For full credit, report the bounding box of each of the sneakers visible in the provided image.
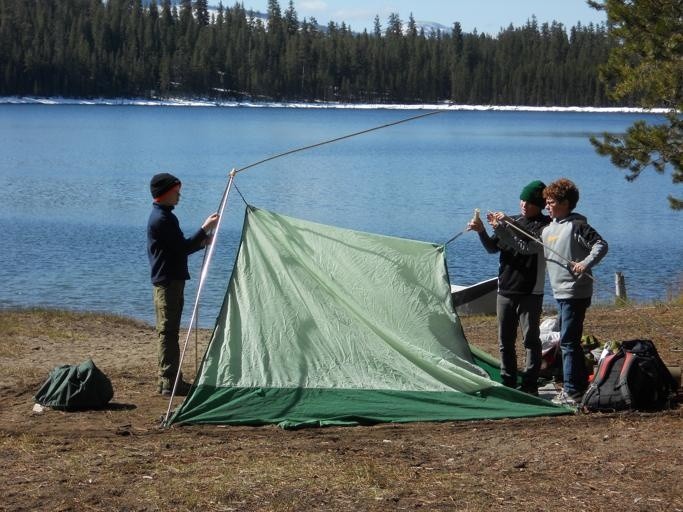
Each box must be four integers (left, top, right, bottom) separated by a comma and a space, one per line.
517, 385, 539, 396
551, 390, 581, 405
157, 380, 193, 396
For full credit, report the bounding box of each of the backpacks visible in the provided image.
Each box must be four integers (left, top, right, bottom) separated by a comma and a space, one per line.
582, 348, 661, 411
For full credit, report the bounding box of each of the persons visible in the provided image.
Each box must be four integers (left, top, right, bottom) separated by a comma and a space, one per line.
485, 178, 609, 405
464, 180, 546, 397
147, 172, 218, 396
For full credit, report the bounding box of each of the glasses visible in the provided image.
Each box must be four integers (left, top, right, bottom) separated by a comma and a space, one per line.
544, 201, 555, 207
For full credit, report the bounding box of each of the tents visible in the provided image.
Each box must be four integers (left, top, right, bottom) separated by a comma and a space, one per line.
167, 203, 575, 424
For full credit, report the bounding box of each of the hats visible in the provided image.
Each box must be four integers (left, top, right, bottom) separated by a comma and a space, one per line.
150, 172, 181, 203
520, 179, 547, 209
